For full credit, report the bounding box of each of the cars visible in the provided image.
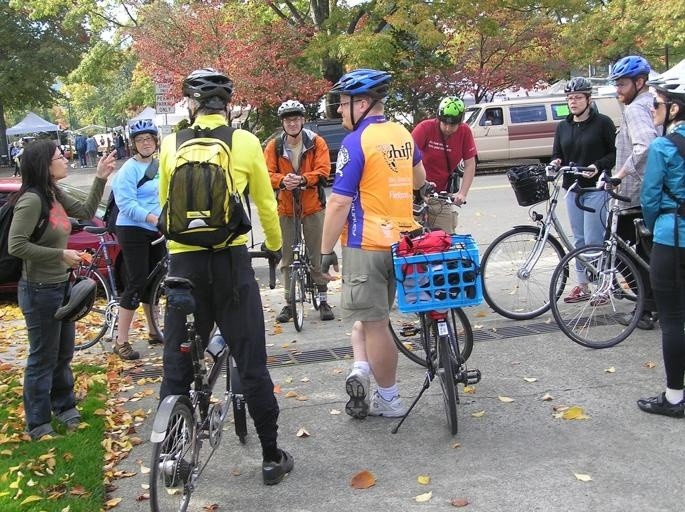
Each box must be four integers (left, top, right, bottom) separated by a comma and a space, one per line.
17, 136, 38, 147
61, 143, 78, 159
0, 179, 129, 302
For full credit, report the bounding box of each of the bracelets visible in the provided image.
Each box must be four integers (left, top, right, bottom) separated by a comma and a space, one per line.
302, 176, 305, 189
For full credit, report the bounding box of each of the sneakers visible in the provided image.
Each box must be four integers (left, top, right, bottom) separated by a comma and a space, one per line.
66, 417, 81, 433
369, 390, 407, 418
276, 305, 293, 323
616, 309, 654, 330
589, 288, 610, 306
32, 431, 55, 440
345, 371, 370, 419
564, 285, 591, 303
320, 303, 334, 321
637, 392, 684, 418
262, 448, 294, 485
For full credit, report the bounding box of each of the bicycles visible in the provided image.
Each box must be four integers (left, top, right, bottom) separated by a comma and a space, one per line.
67, 224, 219, 353
377, 188, 484, 436
274, 180, 321, 331
549, 167, 650, 349
481, 161, 639, 323
413, 190, 467, 230
148, 313, 247, 512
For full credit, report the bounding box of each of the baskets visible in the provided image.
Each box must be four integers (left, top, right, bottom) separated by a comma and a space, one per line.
390, 233, 484, 314
506, 163, 550, 206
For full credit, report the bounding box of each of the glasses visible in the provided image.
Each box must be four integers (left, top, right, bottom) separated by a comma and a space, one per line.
134, 136, 153, 143
565, 96, 586, 101
653, 96, 673, 109
613, 82, 624, 89
283, 116, 301, 124
52, 154, 64, 162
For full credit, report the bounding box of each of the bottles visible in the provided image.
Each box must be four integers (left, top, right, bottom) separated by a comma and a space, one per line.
203, 327, 227, 360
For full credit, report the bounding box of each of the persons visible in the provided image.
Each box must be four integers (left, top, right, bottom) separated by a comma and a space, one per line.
7, 140, 118, 440
603, 56, 661, 328
262, 100, 335, 323
549, 77, 616, 307
157, 67, 293, 486
412, 96, 477, 234
110, 118, 167, 359
635, 73, 685, 420
319, 69, 426, 418
9, 125, 125, 176
487, 111, 502, 125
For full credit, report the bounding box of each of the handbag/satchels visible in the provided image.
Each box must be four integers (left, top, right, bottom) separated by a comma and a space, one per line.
447, 172, 460, 193
103, 190, 119, 234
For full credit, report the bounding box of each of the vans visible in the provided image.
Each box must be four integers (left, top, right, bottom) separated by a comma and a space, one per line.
456, 96, 625, 174
260, 117, 346, 173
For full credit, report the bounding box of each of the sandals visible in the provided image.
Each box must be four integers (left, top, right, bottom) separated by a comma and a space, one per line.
148, 333, 162, 344
114, 342, 138, 360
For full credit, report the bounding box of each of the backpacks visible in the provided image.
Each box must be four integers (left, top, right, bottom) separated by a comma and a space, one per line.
0, 187, 50, 284
156, 124, 253, 310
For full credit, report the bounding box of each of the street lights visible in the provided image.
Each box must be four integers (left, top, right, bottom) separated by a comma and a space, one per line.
103, 113, 109, 135
65, 91, 72, 131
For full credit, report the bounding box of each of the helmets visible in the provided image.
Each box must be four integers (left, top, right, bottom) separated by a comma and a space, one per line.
564, 76, 592, 93
129, 119, 158, 138
53, 275, 97, 323
645, 74, 685, 95
436, 95, 465, 124
183, 68, 234, 101
278, 99, 306, 116
328, 68, 397, 98
606, 55, 650, 81
